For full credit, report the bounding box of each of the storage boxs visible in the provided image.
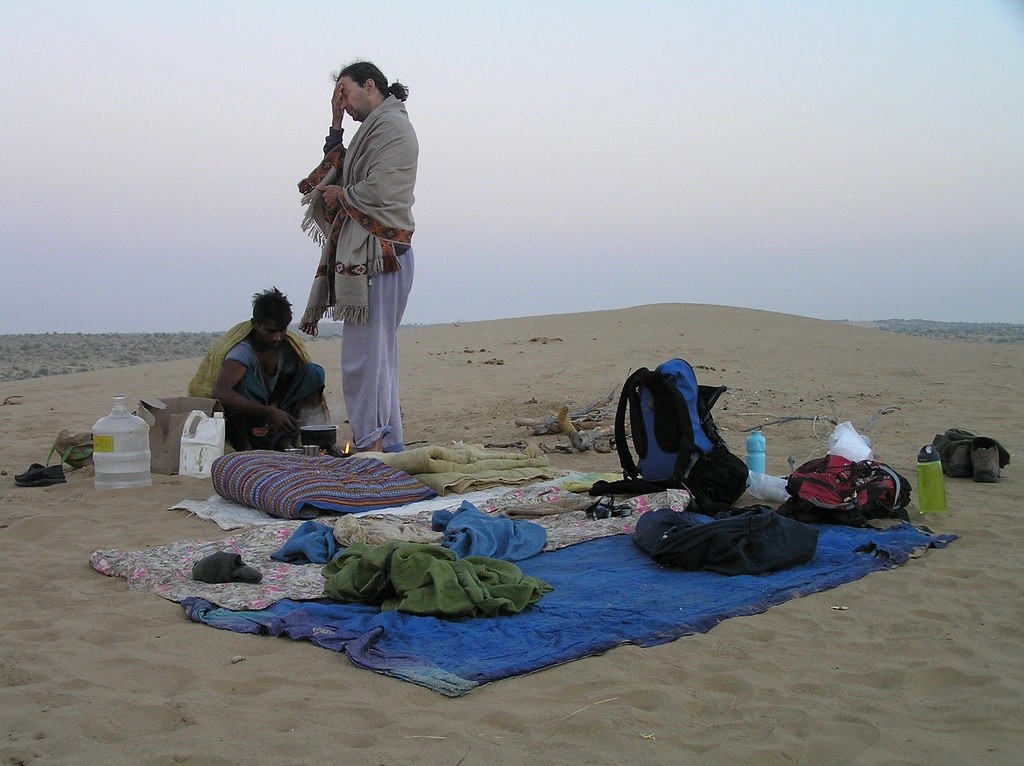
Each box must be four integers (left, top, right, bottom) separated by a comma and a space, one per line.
137, 393, 227, 476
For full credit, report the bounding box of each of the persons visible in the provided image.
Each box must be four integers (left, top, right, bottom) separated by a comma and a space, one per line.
186, 286, 325, 452
315, 60, 419, 457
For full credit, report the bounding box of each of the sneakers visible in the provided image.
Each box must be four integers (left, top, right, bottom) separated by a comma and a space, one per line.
940, 437, 999, 483
14, 463, 66, 487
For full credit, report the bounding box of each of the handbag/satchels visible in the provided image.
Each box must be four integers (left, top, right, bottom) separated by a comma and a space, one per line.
46, 429, 94, 470
673, 444, 749, 517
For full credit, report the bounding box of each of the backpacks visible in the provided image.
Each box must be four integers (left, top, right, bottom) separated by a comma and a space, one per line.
775, 496, 910, 531
786, 455, 912, 511
615, 358, 730, 481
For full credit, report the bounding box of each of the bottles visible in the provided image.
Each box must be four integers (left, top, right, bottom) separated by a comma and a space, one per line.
916, 445, 947, 514
744, 430, 766, 486
178, 410, 227, 481
90, 395, 152, 492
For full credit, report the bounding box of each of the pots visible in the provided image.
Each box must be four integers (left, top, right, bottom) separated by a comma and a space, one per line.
284, 445, 320, 457
299, 424, 338, 448
